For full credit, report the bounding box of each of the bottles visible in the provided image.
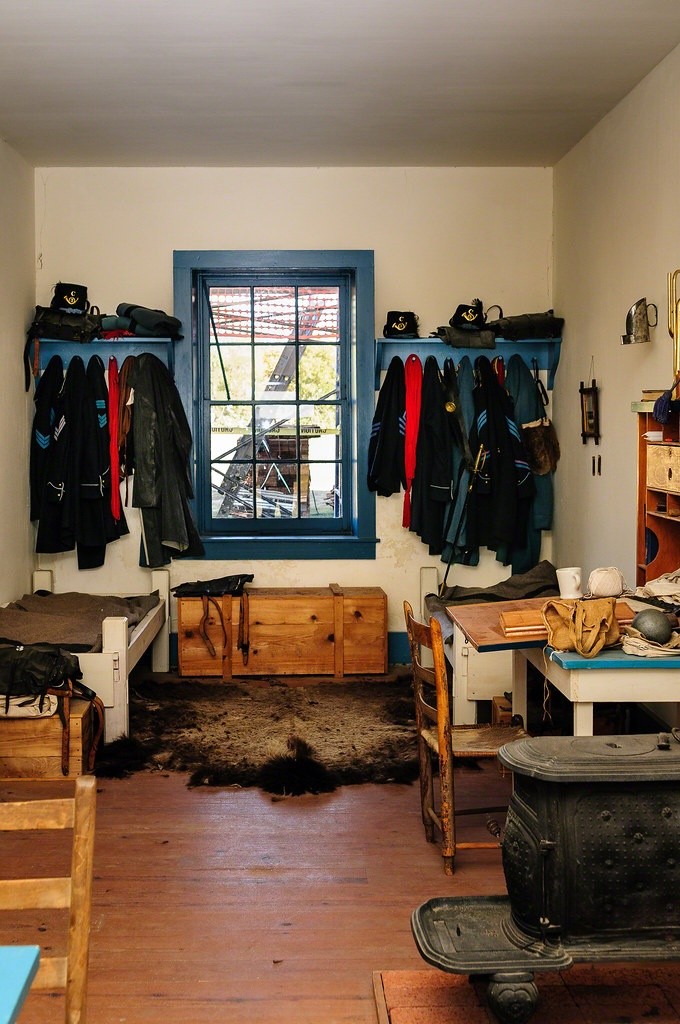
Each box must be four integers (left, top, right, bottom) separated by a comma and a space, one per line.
589, 568, 623, 597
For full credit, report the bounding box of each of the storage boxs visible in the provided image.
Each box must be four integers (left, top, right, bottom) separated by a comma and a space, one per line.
174, 584, 388, 677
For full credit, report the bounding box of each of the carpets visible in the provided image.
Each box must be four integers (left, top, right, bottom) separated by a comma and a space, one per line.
92, 676, 483, 797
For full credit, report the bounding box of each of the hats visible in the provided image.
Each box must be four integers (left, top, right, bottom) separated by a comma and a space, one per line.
383, 310, 420, 338
50, 282, 88, 313
449, 303, 484, 331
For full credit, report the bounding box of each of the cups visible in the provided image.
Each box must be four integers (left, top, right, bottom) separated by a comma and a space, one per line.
556, 567, 583, 600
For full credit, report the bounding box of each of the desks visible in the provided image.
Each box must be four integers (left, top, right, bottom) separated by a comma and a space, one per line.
510, 645, 680, 737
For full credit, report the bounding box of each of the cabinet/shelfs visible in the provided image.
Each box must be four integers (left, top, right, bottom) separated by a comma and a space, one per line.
636, 411, 680, 586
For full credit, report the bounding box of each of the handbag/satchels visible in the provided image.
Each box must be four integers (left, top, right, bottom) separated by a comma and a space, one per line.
27, 303, 106, 342
0, 645, 97, 730
541, 597, 622, 658
483, 305, 564, 340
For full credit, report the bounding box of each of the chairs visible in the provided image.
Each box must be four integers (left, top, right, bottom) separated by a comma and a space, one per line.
0, 775, 97, 1024
404, 600, 532, 876
